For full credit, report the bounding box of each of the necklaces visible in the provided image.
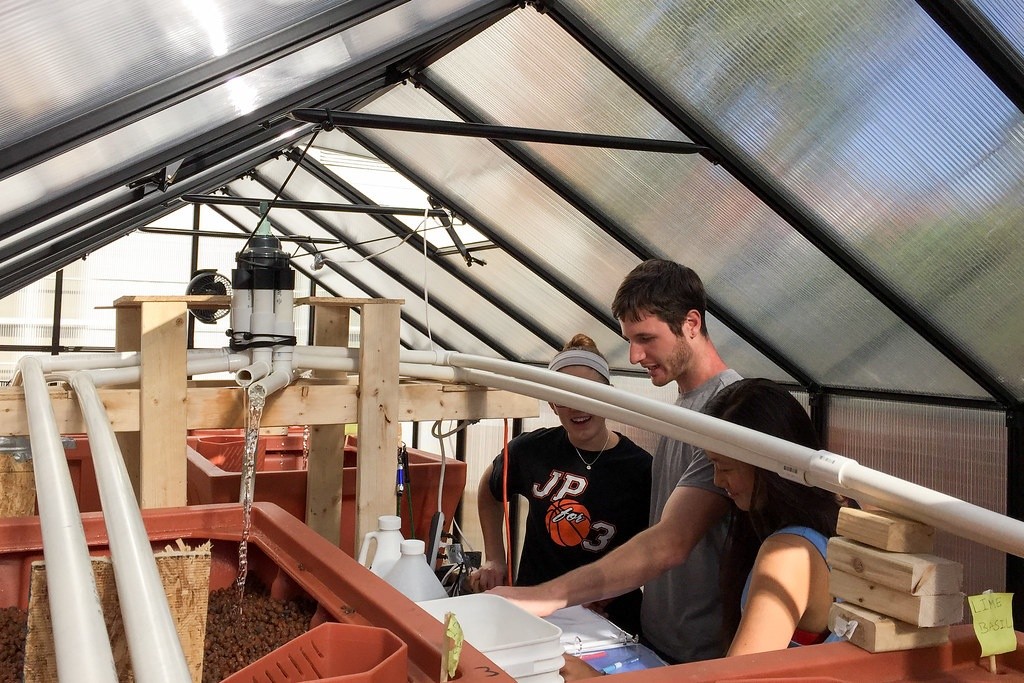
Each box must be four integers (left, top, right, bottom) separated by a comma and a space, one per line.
568, 428, 610, 470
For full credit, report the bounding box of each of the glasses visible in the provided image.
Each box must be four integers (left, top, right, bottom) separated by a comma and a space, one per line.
555, 403, 565, 407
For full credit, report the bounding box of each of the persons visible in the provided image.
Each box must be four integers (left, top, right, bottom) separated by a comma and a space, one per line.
482, 260, 745, 664
470, 333, 653, 642
559, 377, 842, 683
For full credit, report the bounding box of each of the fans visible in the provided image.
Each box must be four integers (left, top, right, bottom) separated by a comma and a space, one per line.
185, 267, 235, 325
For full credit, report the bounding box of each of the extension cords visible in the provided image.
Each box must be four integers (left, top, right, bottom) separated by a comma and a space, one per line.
427, 511, 445, 573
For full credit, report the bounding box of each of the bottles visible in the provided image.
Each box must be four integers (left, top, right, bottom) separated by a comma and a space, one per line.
383, 539, 449, 601
357, 515, 404, 580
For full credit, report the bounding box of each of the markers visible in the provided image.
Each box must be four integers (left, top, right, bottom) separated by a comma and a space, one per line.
600, 656, 640, 675
573, 651, 605, 661
